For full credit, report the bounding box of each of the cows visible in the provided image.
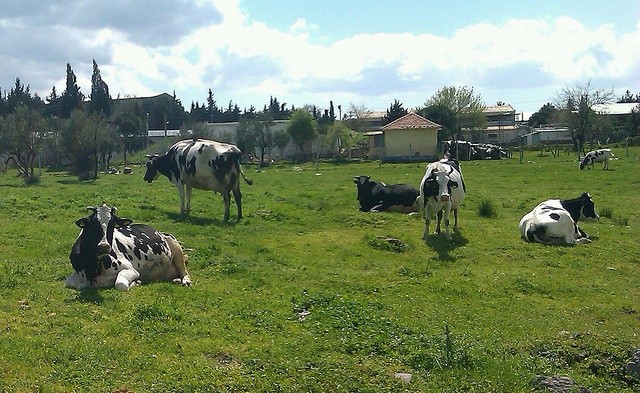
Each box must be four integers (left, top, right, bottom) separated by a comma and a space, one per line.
143, 137, 253, 224
519, 192, 600, 246
419, 157, 467, 240
353, 175, 420, 216
63, 203, 192, 292
579, 150, 610, 171
